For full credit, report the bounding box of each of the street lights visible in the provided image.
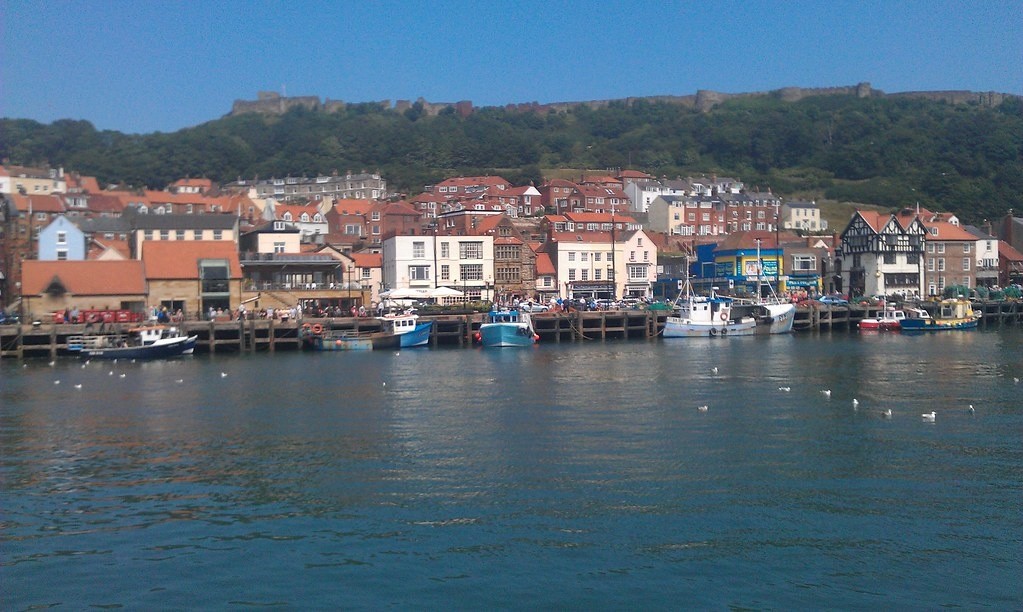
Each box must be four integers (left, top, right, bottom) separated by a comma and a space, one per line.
348, 265, 353, 317
434, 227, 439, 303
773, 213, 779, 298
16, 282, 22, 325
876, 270, 886, 293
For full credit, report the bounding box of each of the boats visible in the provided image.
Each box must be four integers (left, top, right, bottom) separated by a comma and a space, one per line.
302, 315, 433, 350
80, 325, 198, 361
480, 311, 536, 347
900, 300, 982, 330
859, 310, 906, 330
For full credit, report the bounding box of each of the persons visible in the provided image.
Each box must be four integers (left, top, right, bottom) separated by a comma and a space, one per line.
550, 296, 556, 309
563, 296, 570, 313
589, 298, 595, 310
0, 312, 6, 324
580, 296, 585, 311
335, 306, 341, 317
176, 308, 183, 322
875, 290, 919, 301
64, 308, 69, 323
557, 297, 563, 306
260, 304, 302, 320
351, 305, 358, 318
70, 306, 79, 325
157, 305, 165, 323
770, 289, 839, 303
358, 306, 367, 316
493, 297, 535, 312
208, 306, 232, 322
238, 302, 246, 322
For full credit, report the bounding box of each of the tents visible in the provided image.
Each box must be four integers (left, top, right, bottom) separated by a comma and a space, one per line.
378, 287, 464, 314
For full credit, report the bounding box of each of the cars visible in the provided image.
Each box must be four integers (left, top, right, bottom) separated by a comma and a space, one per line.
621, 299, 641, 308
814, 295, 848, 307
593, 299, 620, 310
0, 312, 20, 325
519, 302, 549, 312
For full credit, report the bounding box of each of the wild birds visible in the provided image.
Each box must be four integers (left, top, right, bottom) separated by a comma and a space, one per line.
22, 358, 228, 388
779, 386, 791, 391
921, 410, 936, 418
968, 405, 975, 412
382, 353, 401, 386
820, 389, 831, 395
1012, 377, 1019, 382
880, 408, 891, 415
697, 405, 708, 410
852, 398, 859, 404
710, 366, 719, 372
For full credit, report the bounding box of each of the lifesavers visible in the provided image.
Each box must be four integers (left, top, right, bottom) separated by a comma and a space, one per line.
722, 328, 728, 334
720, 312, 728, 321
55, 310, 131, 324
313, 323, 323, 334
930, 319, 936, 326
710, 327, 718, 336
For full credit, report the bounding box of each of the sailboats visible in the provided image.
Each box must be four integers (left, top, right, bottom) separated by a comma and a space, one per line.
663, 239, 796, 337
